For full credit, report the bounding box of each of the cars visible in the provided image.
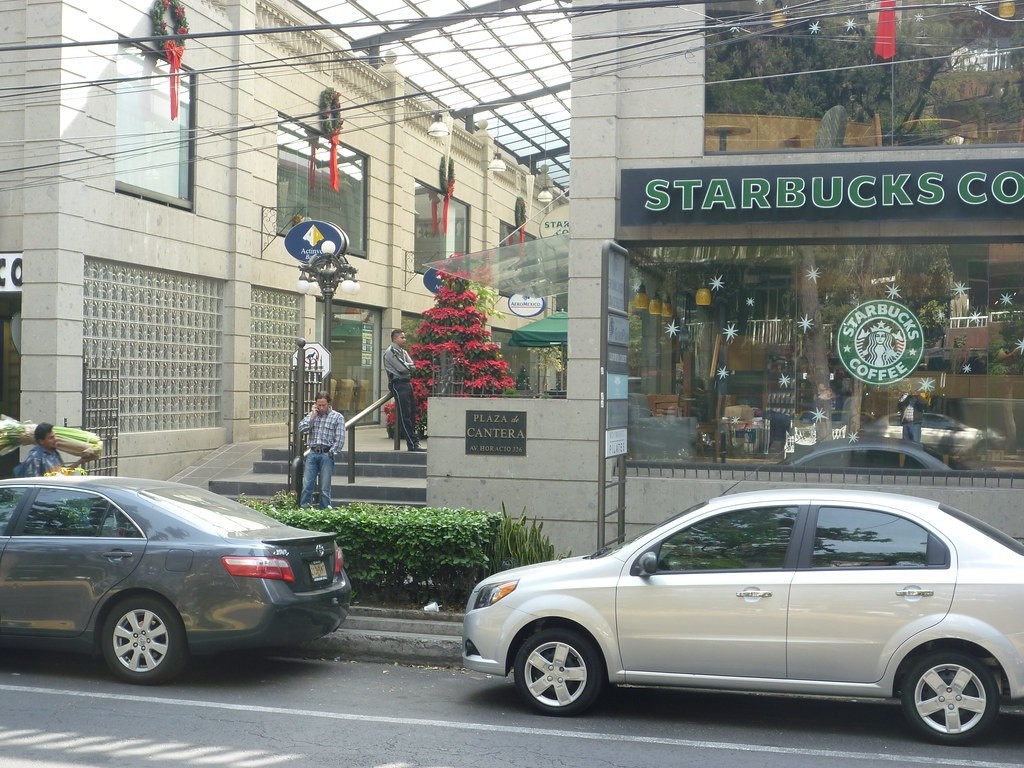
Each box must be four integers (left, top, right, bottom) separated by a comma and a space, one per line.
628, 405, 699, 461
860, 411, 1009, 463
784, 442, 997, 480
0, 476, 352, 680
456, 489, 1024, 746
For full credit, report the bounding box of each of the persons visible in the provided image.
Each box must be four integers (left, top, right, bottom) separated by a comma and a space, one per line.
22, 422, 87, 477
897, 390, 926, 441
383, 329, 427, 452
298, 391, 345, 510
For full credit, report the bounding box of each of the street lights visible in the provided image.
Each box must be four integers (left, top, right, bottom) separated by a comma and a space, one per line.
297, 239, 359, 397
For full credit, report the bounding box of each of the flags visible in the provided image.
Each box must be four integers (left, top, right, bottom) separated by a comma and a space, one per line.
875, 0, 896, 59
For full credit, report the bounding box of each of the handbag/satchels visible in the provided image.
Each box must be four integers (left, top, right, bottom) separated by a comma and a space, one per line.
12, 448, 43, 478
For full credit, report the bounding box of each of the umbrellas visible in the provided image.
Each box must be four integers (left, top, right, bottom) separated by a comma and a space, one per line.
508, 308, 568, 391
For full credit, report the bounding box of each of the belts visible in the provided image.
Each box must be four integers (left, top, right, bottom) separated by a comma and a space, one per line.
311, 446, 331, 454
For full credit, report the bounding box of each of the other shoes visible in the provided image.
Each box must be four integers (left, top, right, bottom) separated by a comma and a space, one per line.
408, 445, 427, 452
